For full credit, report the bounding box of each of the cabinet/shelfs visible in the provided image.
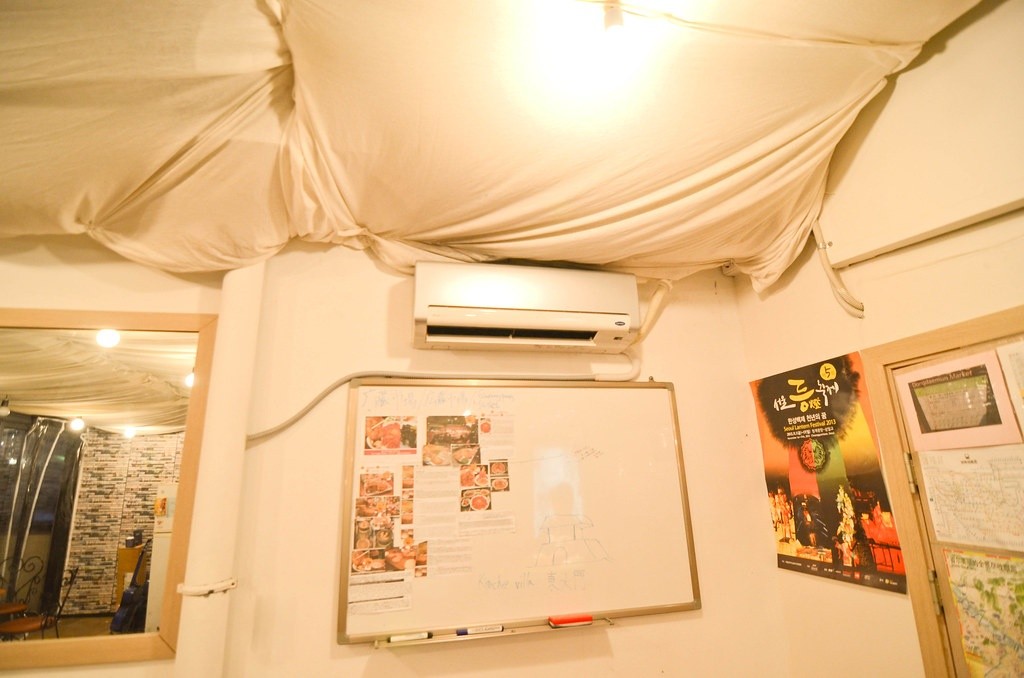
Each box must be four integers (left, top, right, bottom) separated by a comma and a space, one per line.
116, 542, 146, 606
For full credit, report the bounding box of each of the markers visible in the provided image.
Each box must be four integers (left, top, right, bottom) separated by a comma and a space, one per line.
387, 631, 433, 643
456, 625, 504, 636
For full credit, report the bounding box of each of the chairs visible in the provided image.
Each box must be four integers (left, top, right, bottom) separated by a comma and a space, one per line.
0, 552, 79, 640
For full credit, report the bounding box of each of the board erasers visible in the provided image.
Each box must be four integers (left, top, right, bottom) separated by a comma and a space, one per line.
548, 614, 594, 629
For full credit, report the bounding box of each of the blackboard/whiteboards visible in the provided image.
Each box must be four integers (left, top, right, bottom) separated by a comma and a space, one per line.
336, 378, 702, 644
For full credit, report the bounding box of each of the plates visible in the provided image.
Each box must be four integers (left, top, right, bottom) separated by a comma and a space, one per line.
367, 436, 402, 450
364, 480, 392, 496
352, 563, 371, 571
370, 517, 391, 531
461, 469, 508, 511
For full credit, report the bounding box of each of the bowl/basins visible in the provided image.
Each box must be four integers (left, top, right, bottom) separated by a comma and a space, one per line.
356, 538, 370, 549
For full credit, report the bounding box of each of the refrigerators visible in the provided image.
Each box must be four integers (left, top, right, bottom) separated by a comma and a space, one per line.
144, 481, 179, 632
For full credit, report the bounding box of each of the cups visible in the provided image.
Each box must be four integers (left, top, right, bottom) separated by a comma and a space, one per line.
125, 539, 134, 548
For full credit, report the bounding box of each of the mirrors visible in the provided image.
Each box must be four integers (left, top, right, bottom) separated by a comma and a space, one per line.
0, 305, 216, 672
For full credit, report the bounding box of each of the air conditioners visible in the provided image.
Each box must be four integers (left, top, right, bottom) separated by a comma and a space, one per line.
414, 261, 669, 353
